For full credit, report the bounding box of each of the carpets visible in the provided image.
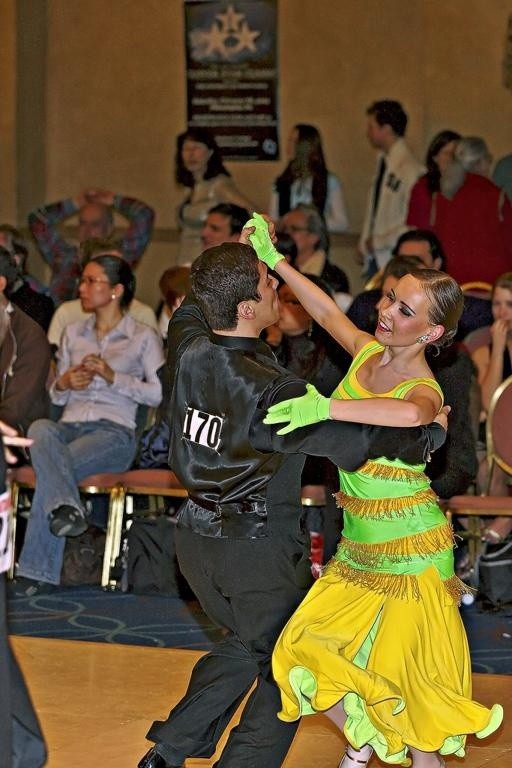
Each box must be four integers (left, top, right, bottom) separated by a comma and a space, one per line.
8, 633, 512, 768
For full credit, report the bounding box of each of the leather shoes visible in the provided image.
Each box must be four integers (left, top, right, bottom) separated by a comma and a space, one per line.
138, 751, 168, 767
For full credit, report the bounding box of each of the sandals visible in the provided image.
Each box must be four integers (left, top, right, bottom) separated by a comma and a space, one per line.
480, 516, 511, 545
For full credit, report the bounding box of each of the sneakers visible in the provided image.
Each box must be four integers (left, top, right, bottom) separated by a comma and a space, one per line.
11, 579, 45, 596
50, 506, 89, 538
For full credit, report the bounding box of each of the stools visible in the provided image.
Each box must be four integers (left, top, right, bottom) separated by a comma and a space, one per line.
14, 465, 328, 594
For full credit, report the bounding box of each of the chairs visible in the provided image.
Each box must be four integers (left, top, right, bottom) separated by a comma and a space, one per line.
445, 375, 512, 528
462, 325, 494, 356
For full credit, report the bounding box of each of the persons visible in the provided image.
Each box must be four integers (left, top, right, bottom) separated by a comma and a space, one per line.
403, 127, 464, 231
45, 236, 161, 572
462, 270, 512, 541
428, 135, 512, 294
278, 202, 350, 294
354, 254, 482, 572
0, 223, 58, 355
0, 419, 48, 768
344, 228, 495, 345
134, 213, 452, 768
352, 99, 429, 293
261, 230, 335, 303
242, 210, 504, 768
5, 253, 167, 601
267, 122, 351, 265
157, 125, 258, 339
136, 265, 193, 471
264, 272, 352, 398
27, 184, 156, 305
0, 244, 53, 468
199, 202, 251, 251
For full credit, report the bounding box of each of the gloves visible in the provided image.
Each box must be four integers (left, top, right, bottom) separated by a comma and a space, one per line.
263, 381, 331, 436
242, 210, 285, 270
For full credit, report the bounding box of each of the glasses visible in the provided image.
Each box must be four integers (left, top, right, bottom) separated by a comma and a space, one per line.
287, 224, 309, 232
76, 275, 116, 285
279, 294, 300, 305
